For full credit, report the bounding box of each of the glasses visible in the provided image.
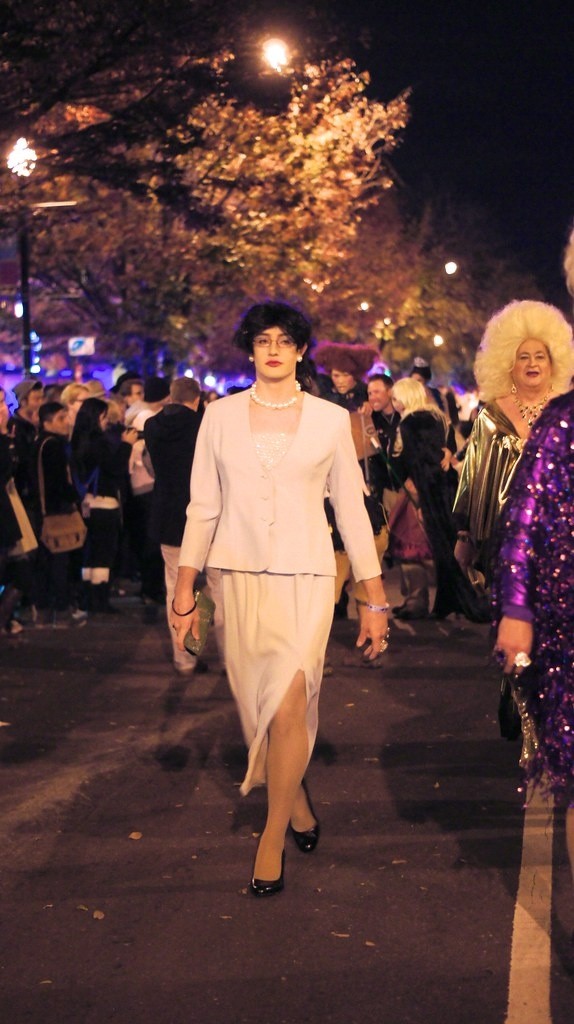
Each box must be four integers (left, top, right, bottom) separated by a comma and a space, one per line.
252, 333, 295, 348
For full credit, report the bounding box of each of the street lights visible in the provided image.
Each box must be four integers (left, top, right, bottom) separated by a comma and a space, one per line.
6, 136, 39, 383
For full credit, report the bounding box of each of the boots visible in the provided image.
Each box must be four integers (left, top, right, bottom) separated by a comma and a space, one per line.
391, 564, 430, 620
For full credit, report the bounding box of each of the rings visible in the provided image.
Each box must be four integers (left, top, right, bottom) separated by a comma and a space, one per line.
380, 640, 388, 652
515, 651, 531, 669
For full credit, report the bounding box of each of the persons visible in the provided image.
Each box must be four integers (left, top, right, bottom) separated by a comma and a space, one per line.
169, 303, 392, 900
490, 231, 574, 879
450, 297, 574, 740
2, 344, 525, 676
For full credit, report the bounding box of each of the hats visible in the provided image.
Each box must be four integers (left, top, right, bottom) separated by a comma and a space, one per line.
13, 379, 40, 403
144, 377, 170, 403
109, 372, 142, 393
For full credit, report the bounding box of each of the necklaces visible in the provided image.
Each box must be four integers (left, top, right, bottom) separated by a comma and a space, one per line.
515, 391, 550, 427
383, 410, 396, 426
250, 379, 302, 409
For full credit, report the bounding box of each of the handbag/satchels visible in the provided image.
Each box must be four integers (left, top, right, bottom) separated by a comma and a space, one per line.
41, 511, 86, 552
468, 566, 491, 608
128, 438, 156, 496
496, 671, 538, 773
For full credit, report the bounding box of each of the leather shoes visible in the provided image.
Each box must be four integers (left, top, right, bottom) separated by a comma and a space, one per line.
250, 850, 287, 897
290, 780, 320, 852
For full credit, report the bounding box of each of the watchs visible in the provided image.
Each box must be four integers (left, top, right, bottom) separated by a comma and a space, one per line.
456, 535, 471, 543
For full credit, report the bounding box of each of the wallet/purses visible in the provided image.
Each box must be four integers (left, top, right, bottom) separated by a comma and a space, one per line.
182, 591, 215, 655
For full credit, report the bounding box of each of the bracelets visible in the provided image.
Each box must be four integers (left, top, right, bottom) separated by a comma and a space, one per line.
170, 597, 197, 616
365, 601, 391, 613
